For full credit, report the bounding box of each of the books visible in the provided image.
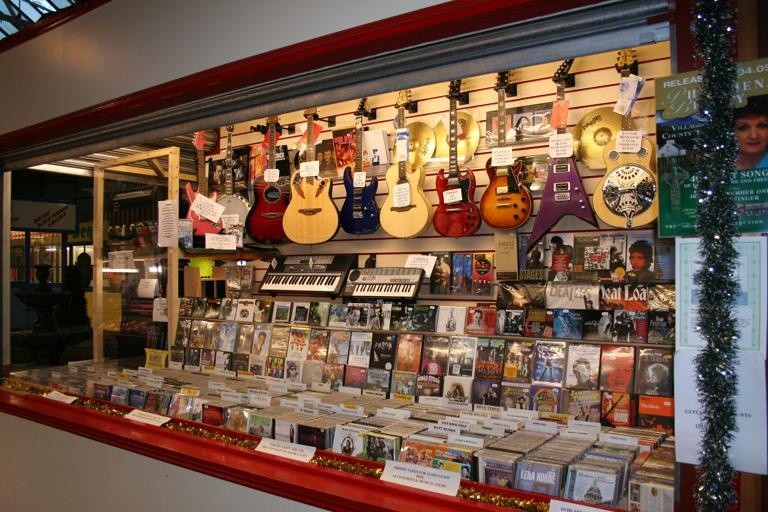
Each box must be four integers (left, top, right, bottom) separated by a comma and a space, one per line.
2, 230, 676, 512
208, 127, 390, 199
654, 57, 768, 235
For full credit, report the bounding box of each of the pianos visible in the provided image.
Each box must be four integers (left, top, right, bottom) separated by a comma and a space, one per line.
343, 267, 425, 303
257, 254, 354, 297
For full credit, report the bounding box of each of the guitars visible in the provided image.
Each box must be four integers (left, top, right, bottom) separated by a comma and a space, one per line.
379, 88, 434, 239
593, 51, 659, 228
282, 106, 340, 245
432, 78, 482, 238
479, 69, 533, 232
338, 95, 380, 235
245, 114, 292, 245
185, 106, 251, 246
525, 58, 601, 254
